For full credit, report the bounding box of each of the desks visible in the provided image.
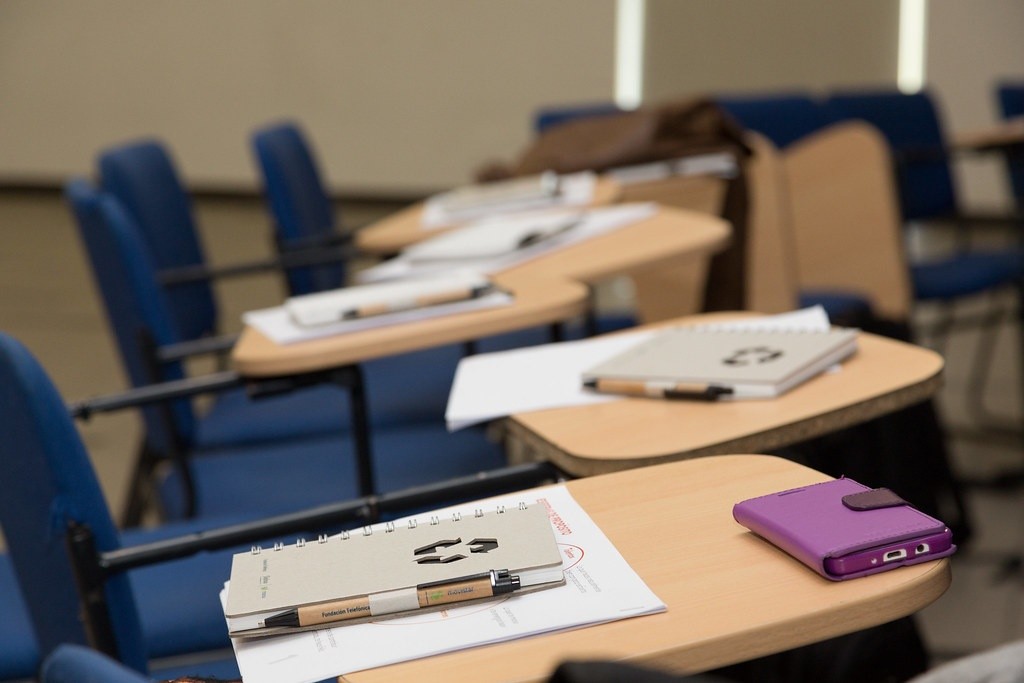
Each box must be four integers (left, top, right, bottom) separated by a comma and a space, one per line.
485, 202, 730, 288
357, 176, 623, 255
501, 313, 947, 478
336, 452, 955, 683
232, 271, 589, 375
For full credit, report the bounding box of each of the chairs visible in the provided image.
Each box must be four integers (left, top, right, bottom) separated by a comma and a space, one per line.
839, 87, 1024, 437
248, 121, 399, 297
0, 553, 153, 683
94, 137, 463, 455
0, 332, 559, 683
65, 175, 501, 534
719, 91, 833, 157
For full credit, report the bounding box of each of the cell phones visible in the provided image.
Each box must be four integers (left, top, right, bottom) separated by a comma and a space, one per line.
732, 476, 957, 582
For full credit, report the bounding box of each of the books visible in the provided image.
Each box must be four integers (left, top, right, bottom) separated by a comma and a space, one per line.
580, 316, 860, 405
224, 501, 567, 639
283, 173, 579, 327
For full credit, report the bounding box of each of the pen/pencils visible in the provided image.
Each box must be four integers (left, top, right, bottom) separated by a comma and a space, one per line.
259, 568, 522, 631
585, 380, 734, 400
341, 281, 495, 321
